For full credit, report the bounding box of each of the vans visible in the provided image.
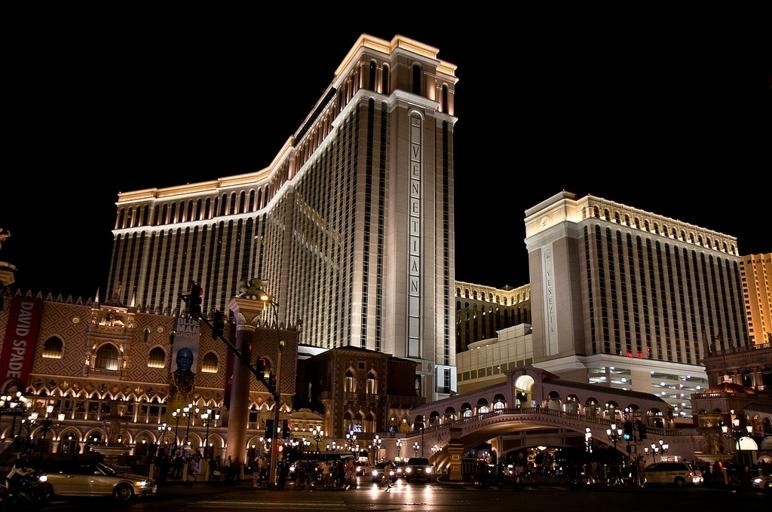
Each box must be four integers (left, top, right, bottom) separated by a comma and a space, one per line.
642, 460, 704, 488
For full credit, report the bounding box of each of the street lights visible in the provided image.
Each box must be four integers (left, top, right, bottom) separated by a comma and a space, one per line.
0, 390, 67, 440
396, 440, 403, 459
157, 401, 221, 449
252, 287, 282, 487
312, 423, 324, 453
605, 423, 624, 451
412, 441, 420, 458
372, 434, 384, 464
643, 439, 669, 463
721, 418, 754, 504
345, 428, 358, 451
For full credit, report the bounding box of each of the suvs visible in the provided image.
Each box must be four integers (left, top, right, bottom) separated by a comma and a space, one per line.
404, 456, 433, 483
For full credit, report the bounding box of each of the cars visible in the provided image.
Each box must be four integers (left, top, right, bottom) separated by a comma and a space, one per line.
28, 461, 159, 502
355, 460, 405, 484
751, 473, 772, 490
